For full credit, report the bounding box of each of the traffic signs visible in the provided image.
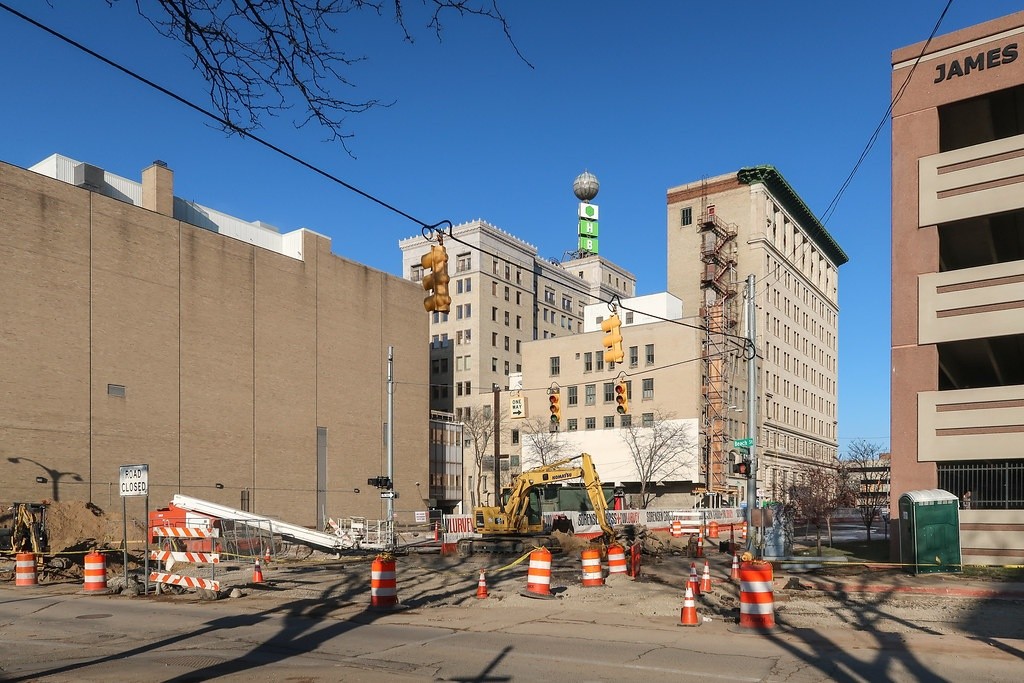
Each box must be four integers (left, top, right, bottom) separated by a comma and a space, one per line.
509, 397, 526, 418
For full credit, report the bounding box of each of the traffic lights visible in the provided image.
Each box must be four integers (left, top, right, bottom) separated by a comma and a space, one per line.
615, 383, 628, 414
600, 313, 626, 363
548, 394, 561, 422
419, 245, 453, 313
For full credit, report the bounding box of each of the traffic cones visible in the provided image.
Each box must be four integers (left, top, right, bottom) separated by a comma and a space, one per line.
686, 560, 702, 597
476, 568, 490, 599
727, 524, 738, 555
266, 547, 271, 565
695, 535, 706, 557
677, 582, 702, 627
731, 551, 743, 583
700, 560, 713, 594
252, 557, 266, 582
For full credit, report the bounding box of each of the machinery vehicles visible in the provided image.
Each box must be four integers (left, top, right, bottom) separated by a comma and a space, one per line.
150, 496, 355, 562
454, 452, 619, 556
1, 502, 48, 581
